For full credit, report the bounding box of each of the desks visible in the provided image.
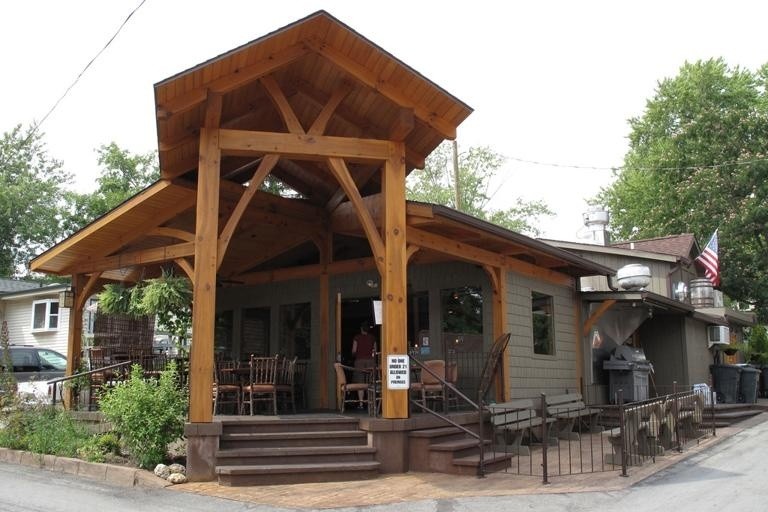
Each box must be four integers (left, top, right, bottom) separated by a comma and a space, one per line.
361, 367, 421, 413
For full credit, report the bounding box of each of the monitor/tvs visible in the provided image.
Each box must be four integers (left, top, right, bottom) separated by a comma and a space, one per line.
371, 297, 383, 327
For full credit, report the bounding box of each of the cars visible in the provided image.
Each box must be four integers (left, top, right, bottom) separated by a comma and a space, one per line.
154, 338, 169, 350
0, 345, 67, 405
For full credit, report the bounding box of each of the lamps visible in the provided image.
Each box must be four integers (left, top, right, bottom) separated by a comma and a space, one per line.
58, 285, 74, 308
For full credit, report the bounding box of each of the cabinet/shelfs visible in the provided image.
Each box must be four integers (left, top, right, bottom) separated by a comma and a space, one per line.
602, 360, 649, 407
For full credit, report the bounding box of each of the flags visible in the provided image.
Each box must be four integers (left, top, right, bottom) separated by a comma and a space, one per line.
695, 230, 720, 287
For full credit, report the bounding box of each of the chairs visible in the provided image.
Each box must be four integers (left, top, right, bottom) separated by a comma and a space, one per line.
90, 354, 312, 410
447, 358, 460, 410
410, 359, 445, 413
334, 362, 369, 414
371, 351, 405, 415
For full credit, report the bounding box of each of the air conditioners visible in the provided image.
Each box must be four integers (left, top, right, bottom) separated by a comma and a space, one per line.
706, 325, 730, 346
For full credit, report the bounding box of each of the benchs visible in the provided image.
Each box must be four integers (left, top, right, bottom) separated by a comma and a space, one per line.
485, 388, 705, 467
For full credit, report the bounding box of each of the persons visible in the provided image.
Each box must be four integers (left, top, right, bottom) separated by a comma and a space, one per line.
349, 320, 379, 411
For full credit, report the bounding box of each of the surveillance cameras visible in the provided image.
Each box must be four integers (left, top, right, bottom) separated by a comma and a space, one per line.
366, 280, 374, 289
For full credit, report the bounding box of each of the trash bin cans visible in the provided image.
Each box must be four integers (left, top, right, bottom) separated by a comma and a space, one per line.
710, 364, 743, 404
603, 345, 650, 405
736, 366, 762, 403
